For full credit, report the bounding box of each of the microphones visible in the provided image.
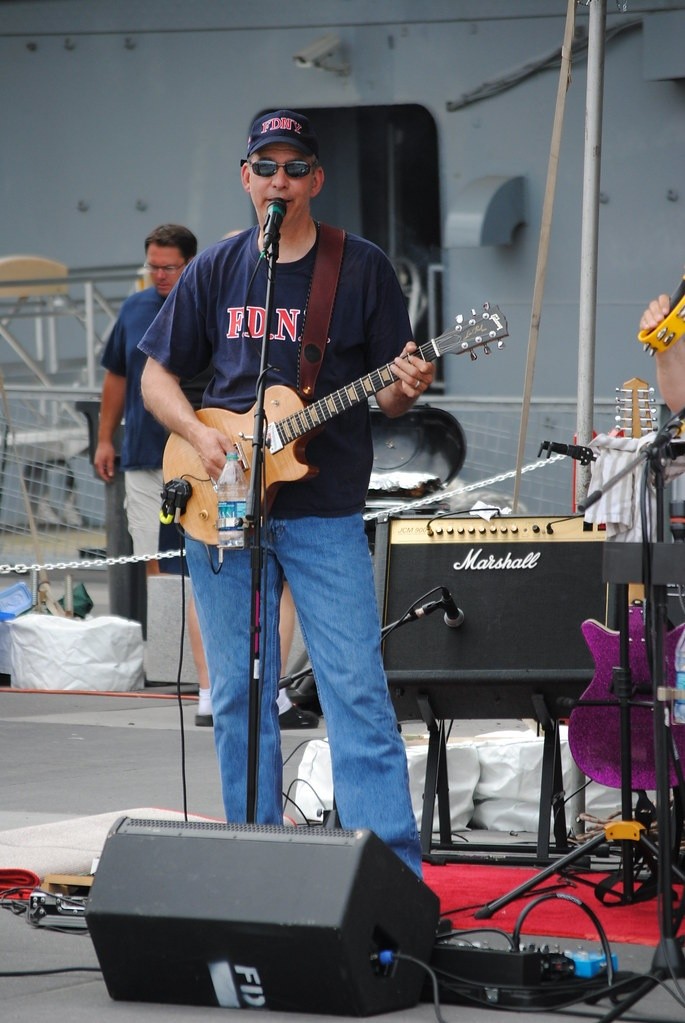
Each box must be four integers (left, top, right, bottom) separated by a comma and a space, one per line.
441, 585, 464, 627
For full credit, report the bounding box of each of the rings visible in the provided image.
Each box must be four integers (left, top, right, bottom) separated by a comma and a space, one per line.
415, 381, 420, 388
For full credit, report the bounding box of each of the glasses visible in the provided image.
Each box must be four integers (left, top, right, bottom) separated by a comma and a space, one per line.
144, 257, 191, 275
248, 160, 316, 177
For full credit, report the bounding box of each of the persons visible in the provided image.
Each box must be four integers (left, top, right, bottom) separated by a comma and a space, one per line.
181, 229, 319, 733
94, 222, 198, 576
135, 109, 436, 879
640, 294, 685, 416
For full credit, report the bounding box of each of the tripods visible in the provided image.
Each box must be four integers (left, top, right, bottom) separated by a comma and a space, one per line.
474, 414, 685, 1023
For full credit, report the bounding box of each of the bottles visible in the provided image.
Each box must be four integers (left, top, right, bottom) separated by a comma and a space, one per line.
215, 451, 249, 550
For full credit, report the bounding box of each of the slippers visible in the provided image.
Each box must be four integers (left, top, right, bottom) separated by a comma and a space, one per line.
195, 715, 213, 726
278, 705, 320, 729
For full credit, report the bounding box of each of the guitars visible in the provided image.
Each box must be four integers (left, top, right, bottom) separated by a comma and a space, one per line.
566, 377, 685, 793
160, 301, 514, 546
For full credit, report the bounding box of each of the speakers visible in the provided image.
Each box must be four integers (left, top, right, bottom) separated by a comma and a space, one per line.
83, 815, 440, 1018
374, 511, 619, 721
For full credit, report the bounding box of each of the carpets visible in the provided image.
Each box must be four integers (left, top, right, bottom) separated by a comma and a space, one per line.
0, 805, 685, 946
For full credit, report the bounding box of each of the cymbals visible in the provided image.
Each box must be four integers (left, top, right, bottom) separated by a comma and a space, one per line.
641, 342, 658, 357
676, 304, 685, 323
656, 327, 676, 347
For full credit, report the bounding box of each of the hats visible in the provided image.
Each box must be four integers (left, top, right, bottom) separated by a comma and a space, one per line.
240, 109, 319, 168
